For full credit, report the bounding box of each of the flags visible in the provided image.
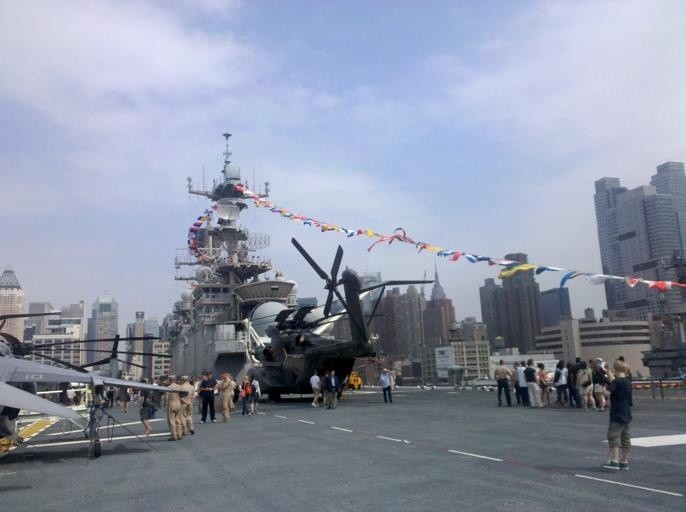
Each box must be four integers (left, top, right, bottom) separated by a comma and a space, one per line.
185, 201, 219, 263
236, 185, 686, 295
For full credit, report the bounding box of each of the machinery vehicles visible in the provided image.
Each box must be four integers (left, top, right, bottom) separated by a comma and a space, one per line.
346, 369, 362, 390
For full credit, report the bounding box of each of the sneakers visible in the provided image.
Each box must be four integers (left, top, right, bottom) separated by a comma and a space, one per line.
620, 462, 629, 470
602, 459, 620, 470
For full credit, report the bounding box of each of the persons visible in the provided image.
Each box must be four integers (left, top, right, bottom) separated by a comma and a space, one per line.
603, 360, 633, 470
494, 356, 635, 409
380, 366, 392, 401
310, 369, 341, 410
93, 372, 262, 442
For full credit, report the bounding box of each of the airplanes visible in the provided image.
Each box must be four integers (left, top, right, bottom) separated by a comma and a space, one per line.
0, 311, 189, 459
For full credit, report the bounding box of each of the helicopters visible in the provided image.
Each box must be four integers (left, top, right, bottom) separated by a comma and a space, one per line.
245, 235, 436, 402
